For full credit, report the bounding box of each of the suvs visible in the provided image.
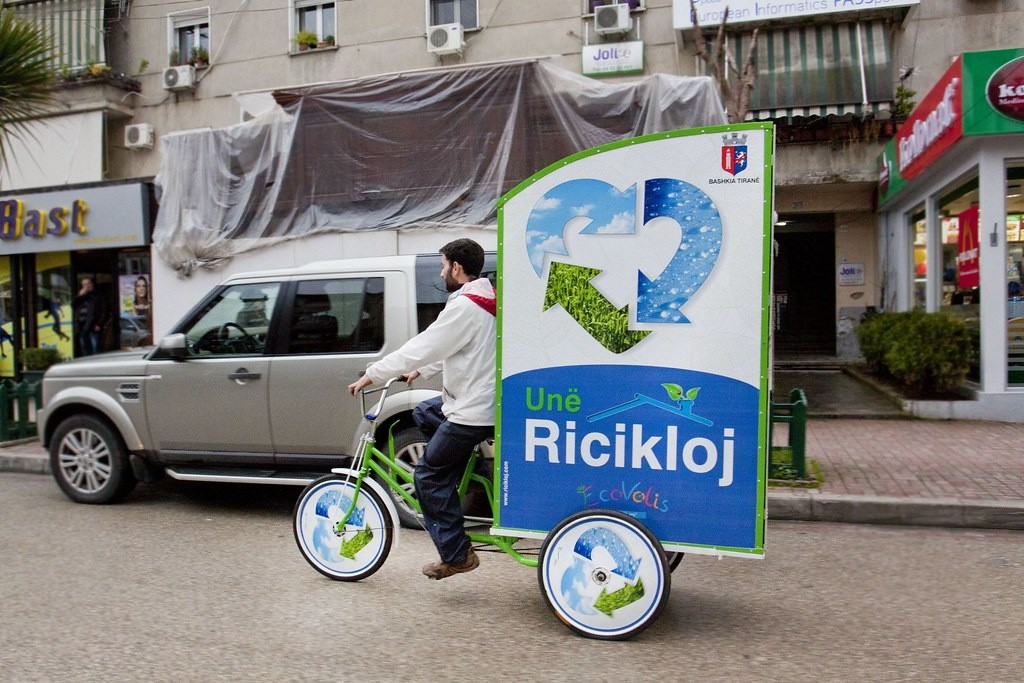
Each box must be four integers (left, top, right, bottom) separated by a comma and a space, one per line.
35, 254, 501, 505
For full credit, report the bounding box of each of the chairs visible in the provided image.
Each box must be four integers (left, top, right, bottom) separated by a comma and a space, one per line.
289, 294, 339, 354
343, 287, 384, 352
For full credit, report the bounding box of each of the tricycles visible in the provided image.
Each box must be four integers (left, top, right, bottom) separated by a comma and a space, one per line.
291, 119, 776, 648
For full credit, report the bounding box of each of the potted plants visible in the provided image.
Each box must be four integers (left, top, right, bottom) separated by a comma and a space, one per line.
289, 30, 318, 52
194, 47, 209, 65
325, 35, 334, 46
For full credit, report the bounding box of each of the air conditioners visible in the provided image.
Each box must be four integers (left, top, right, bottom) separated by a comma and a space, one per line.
124, 122, 154, 150
161, 64, 197, 92
426, 22, 465, 55
594, 3, 633, 34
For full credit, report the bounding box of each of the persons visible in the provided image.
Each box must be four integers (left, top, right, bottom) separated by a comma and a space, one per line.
349, 238, 497, 579
132, 276, 150, 316
72, 278, 105, 357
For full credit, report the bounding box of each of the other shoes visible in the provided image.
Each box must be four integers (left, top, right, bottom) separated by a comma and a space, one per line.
423, 546, 479, 578
